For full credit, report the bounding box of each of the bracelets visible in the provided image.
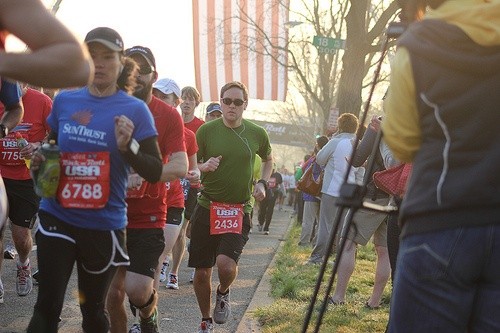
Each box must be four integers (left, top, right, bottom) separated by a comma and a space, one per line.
121, 138, 140, 160
258, 179, 268, 190
40, 141, 45, 145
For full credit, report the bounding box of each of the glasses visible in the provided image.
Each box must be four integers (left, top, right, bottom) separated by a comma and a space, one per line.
222, 97, 246, 106
138, 65, 151, 74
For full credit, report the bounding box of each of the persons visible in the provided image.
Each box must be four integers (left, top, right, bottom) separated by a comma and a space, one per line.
258, 163, 286, 235
371, 115, 413, 287
129, 78, 224, 323
105, 46, 189, 333
274, 165, 296, 209
26, 27, 163, 333
294, 113, 367, 268
187, 81, 273, 333
0, 0, 94, 304
325, 85, 391, 309
381, 0, 500, 333
250, 154, 262, 227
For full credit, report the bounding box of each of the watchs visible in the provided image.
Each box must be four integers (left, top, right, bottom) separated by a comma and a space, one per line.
0, 123, 8, 138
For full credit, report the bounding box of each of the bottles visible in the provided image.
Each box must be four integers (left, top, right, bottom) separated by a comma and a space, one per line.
15, 132, 35, 168
35, 139, 61, 199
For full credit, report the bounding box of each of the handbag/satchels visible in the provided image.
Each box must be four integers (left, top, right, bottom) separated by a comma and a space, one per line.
296, 161, 322, 196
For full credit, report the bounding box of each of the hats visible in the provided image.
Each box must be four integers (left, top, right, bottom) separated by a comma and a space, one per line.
208, 106, 223, 114
124, 46, 156, 71
84, 27, 125, 52
151, 78, 180, 100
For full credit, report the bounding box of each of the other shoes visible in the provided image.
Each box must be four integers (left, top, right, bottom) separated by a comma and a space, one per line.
258, 224, 262, 233
129, 324, 140, 333
264, 231, 268, 235
189, 272, 193, 282
329, 297, 343, 305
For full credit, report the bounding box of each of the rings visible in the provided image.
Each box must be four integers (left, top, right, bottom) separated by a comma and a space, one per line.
31, 153, 32, 156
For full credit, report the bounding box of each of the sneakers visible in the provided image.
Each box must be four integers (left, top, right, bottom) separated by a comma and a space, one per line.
199, 319, 211, 333
3, 245, 18, 259
138, 307, 158, 333
213, 284, 230, 324
166, 271, 178, 290
0, 287, 4, 303
159, 261, 170, 282
16, 262, 31, 295
